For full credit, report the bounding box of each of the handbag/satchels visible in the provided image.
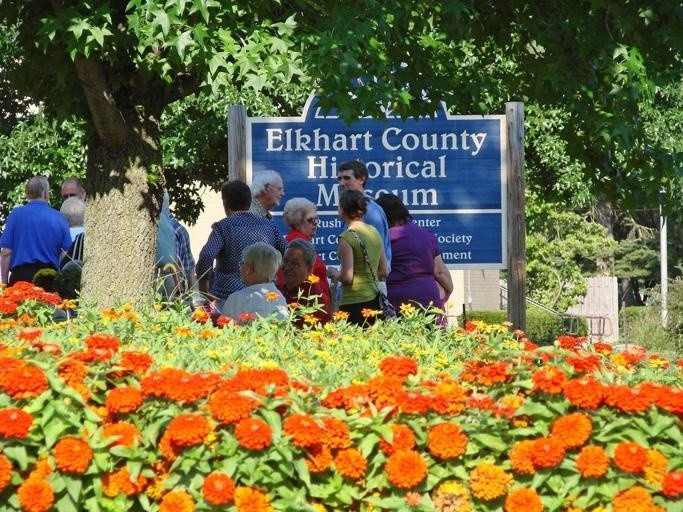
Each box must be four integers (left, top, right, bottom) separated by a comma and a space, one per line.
379, 293, 397, 320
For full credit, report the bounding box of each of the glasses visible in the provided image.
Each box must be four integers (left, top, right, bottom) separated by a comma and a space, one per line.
62, 193, 77, 198
239, 261, 246, 266
338, 175, 350, 180
306, 217, 320, 225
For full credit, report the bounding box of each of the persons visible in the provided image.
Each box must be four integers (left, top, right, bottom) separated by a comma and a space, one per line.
195, 171, 330, 329
1, 177, 86, 285
325, 161, 452, 330
156, 189, 195, 301
